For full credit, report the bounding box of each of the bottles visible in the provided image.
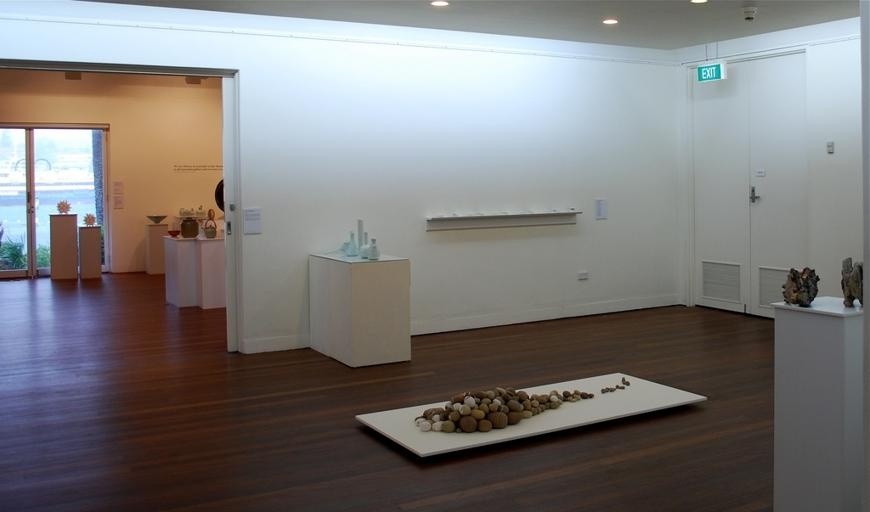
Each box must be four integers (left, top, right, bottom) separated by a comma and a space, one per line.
367, 238, 380, 260
345, 232, 359, 256
359, 232, 370, 259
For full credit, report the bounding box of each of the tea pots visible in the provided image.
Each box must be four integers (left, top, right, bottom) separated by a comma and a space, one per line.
201, 219, 217, 239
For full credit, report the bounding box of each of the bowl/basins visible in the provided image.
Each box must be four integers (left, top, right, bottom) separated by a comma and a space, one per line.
146, 215, 167, 224
168, 230, 181, 238
